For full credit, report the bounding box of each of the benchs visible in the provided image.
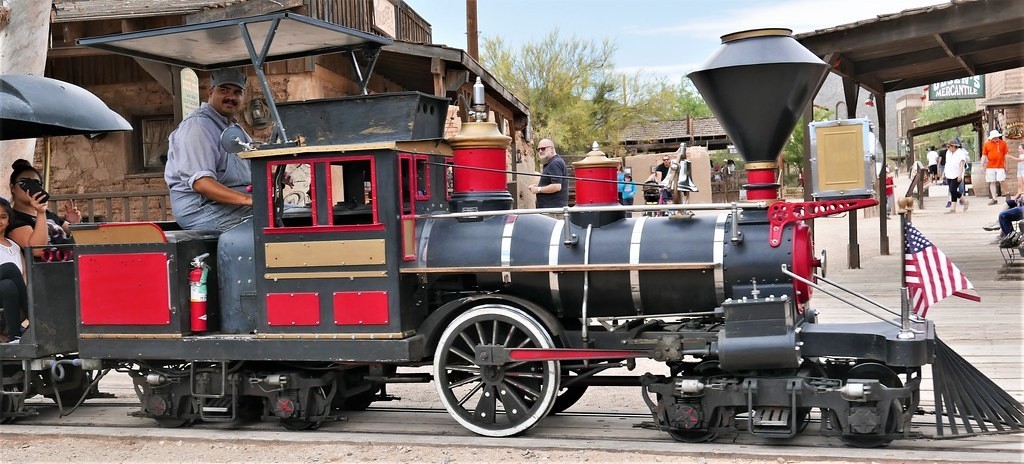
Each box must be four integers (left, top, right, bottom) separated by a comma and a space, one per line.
68, 222, 224, 331
998, 220, 1024, 281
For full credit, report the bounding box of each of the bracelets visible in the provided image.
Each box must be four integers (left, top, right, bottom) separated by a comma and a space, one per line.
538, 187, 541, 193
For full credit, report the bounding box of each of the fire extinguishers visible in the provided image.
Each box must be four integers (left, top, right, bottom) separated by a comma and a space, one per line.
189, 252, 212, 333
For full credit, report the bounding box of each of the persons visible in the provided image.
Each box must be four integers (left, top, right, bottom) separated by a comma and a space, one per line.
983, 191, 1024, 243
895, 163, 899, 178
945, 137, 970, 214
886, 163, 893, 173
1005, 144, 1024, 190
529, 139, 569, 208
164, 67, 252, 230
799, 167, 805, 187
617, 160, 637, 218
0, 159, 81, 341
927, 146, 939, 185
647, 155, 671, 197
886, 170, 896, 209
939, 144, 948, 184
710, 159, 735, 181
980, 130, 1011, 205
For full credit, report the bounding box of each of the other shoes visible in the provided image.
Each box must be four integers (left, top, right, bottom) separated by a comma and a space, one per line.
1005, 192, 1011, 202
990, 236, 1006, 244
963, 200, 969, 212
982, 222, 1001, 231
944, 209, 956, 214
988, 199, 997, 205
6, 333, 22, 343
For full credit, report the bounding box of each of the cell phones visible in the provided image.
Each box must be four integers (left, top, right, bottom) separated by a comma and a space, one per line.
20, 177, 50, 204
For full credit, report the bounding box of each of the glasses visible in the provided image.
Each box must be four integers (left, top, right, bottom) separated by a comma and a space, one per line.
14, 177, 42, 186
662, 158, 670, 161
536, 146, 552, 152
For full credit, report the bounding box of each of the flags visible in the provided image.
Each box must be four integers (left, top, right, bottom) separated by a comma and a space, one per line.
904, 219, 981, 318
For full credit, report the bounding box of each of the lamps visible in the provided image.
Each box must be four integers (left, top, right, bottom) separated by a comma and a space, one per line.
244, 99, 271, 130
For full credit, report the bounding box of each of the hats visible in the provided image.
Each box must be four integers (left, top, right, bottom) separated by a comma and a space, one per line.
988, 129, 1002, 139
210, 67, 247, 90
944, 140, 960, 146
